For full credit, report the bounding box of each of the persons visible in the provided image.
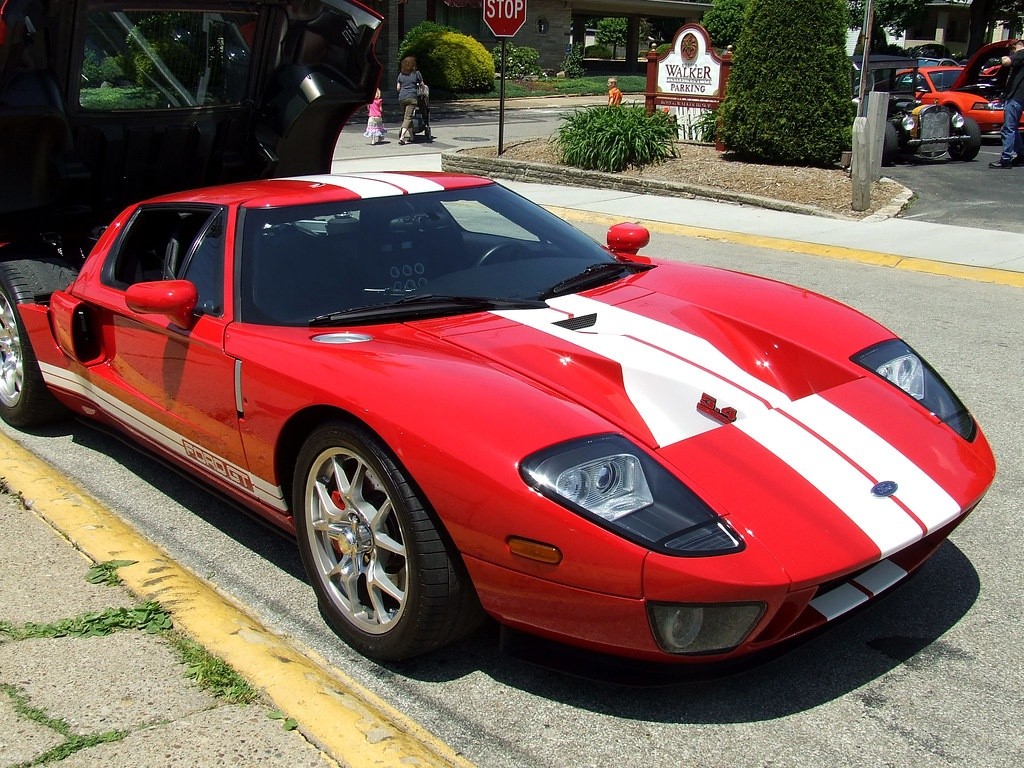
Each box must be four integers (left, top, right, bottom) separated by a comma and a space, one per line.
397, 57, 425, 145
988, 39, 1024, 169
607, 78, 623, 107
363, 88, 387, 145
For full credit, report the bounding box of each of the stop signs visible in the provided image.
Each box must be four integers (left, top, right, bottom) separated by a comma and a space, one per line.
483, 0, 527, 38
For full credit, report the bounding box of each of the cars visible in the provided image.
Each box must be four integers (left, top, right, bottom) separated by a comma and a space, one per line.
902, 56, 960, 82
896, 39, 1024, 134
846, 54, 981, 161
959, 58, 990, 70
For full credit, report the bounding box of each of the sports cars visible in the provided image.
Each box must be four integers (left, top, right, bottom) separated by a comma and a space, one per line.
0, 0, 996, 669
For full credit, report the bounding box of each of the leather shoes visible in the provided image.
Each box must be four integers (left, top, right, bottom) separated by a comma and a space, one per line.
988, 155, 1022, 169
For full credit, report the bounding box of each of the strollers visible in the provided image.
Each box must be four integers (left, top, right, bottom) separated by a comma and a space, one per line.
399, 86, 432, 143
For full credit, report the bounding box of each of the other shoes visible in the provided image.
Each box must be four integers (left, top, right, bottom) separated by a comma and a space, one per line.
409, 139, 415, 144
378, 138, 382, 143
399, 140, 407, 145
371, 140, 375, 145
